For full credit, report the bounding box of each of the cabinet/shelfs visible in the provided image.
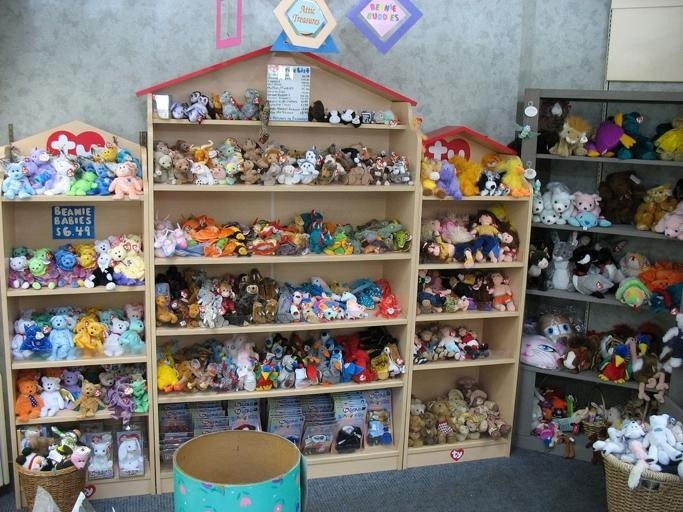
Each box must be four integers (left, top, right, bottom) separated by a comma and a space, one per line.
402, 127, 534, 470
0, 120, 156, 512
136, 30, 423, 498
512, 88, 683, 464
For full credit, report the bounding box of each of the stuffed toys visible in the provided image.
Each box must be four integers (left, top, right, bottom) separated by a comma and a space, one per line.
525, 225, 683, 319
405, 374, 513, 451
152, 207, 415, 260
412, 269, 522, 316
153, 263, 406, 330
8, 301, 147, 363
4, 231, 147, 292
528, 382, 683, 498
516, 303, 683, 405
12, 362, 149, 433
417, 203, 529, 266
410, 321, 493, 367
529, 168, 683, 243
2, 142, 146, 202
15, 419, 147, 484
150, 91, 404, 146
505, 99, 683, 163
416, 149, 533, 204
154, 325, 408, 397
152, 134, 415, 188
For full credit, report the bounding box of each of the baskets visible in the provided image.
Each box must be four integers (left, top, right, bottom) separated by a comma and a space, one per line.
601, 451, 683, 511
15, 462, 86, 512
